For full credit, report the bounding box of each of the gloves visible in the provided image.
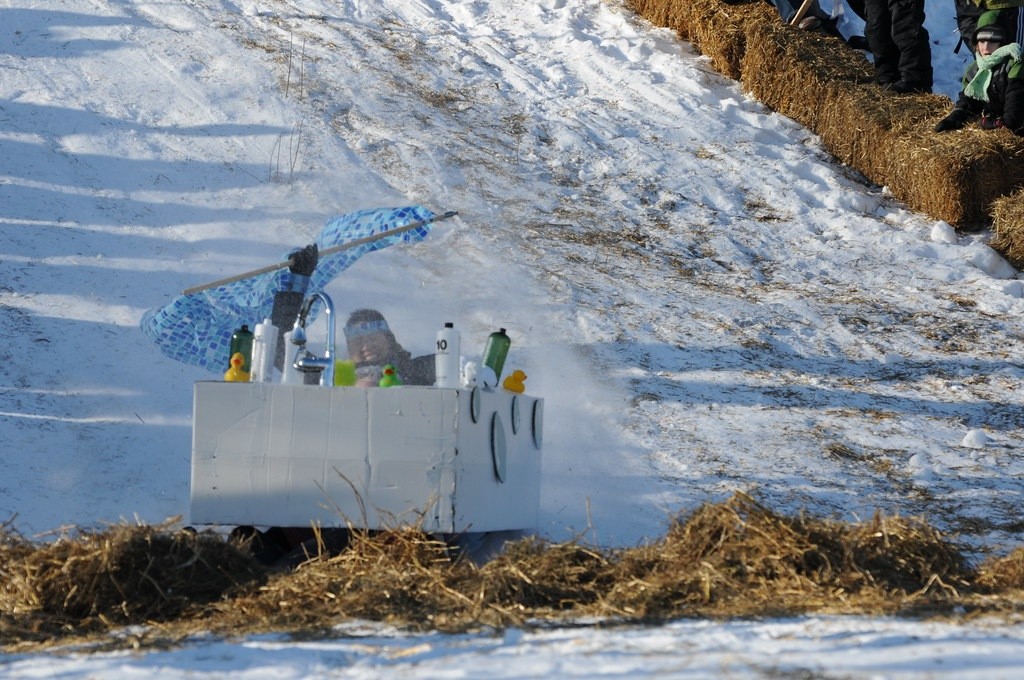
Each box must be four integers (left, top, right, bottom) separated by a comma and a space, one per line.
935, 110, 972, 132
289, 243, 319, 276
981, 108, 1003, 130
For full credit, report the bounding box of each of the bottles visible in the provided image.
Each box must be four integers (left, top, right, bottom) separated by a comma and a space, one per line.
481, 328, 511, 388
435, 322, 460, 388
283, 331, 307, 384
229, 325, 254, 372
250, 318, 279, 383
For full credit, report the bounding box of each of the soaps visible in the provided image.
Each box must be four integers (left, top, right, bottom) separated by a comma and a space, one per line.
335, 360, 355, 386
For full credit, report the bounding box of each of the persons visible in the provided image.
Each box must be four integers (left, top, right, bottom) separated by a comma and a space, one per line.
769, 0, 1024, 132
345, 309, 437, 387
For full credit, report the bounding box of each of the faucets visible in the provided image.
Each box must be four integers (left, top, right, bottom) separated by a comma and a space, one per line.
291, 291, 334, 385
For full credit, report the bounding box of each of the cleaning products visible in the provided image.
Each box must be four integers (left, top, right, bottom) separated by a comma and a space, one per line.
483, 327, 511, 389
436, 322, 461, 387
229, 318, 279, 383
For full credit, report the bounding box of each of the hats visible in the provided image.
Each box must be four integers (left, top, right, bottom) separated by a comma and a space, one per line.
976, 26, 1004, 42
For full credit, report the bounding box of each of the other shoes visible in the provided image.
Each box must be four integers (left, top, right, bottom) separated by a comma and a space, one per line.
798, 16, 821, 31
882, 79, 932, 95
